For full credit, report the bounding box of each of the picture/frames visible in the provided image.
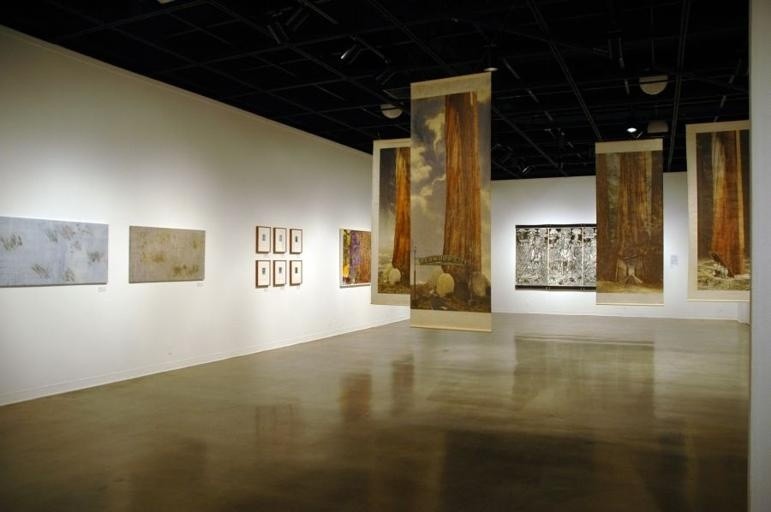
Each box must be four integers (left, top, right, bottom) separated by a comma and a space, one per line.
255, 225, 304, 286
